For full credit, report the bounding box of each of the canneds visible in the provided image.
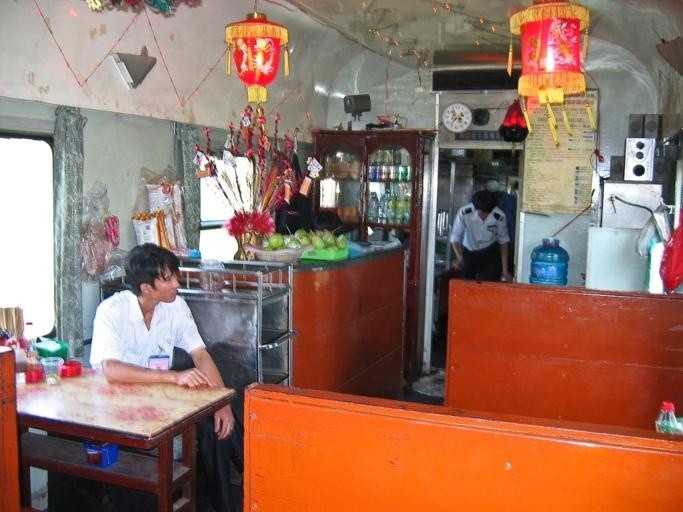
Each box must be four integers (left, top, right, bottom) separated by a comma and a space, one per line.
368, 162, 413, 181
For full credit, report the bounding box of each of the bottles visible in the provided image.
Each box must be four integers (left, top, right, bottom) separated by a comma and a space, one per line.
656, 401, 678, 435
366, 189, 412, 223
21, 320, 46, 386
197, 258, 227, 292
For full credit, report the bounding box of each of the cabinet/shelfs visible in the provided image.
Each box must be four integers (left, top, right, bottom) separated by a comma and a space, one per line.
311, 128, 435, 384
100, 258, 293, 431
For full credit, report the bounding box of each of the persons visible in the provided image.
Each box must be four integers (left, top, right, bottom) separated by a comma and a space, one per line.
448, 191, 512, 281
88, 242, 244, 511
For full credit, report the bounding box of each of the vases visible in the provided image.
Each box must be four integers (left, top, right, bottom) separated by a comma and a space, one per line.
233, 230, 260, 262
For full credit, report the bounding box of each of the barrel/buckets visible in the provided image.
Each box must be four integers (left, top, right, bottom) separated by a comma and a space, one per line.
528, 237, 569, 286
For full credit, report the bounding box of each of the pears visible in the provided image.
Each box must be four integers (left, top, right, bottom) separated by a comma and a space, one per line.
262, 226, 347, 251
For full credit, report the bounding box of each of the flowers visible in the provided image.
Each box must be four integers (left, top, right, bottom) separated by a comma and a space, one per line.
192, 106, 305, 238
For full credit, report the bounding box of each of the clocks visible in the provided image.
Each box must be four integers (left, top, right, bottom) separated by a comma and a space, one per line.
440, 102, 474, 133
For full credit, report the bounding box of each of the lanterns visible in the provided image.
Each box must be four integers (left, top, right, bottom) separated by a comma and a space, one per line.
506, 0, 596, 144
223, 11, 290, 103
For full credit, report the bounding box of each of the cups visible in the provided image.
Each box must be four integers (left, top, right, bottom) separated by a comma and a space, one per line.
40, 356, 64, 386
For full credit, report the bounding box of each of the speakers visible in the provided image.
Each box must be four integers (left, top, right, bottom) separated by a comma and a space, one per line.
628, 114, 643, 136
643, 114, 661, 139
624, 137, 654, 182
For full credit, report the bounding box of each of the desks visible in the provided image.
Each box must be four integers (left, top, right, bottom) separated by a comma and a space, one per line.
16, 365, 238, 511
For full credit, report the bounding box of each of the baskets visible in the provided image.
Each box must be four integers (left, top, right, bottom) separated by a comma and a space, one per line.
246, 245, 349, 263
